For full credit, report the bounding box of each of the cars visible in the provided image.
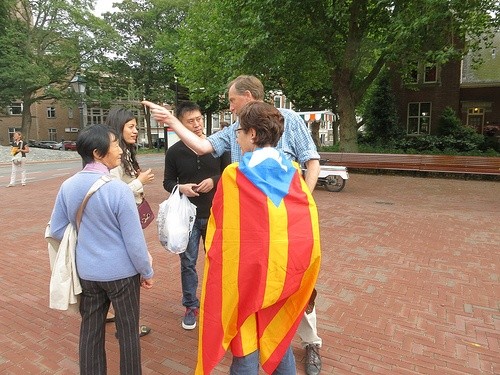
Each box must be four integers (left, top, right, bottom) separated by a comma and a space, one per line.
26, 139, 78, 152
137, 137, 164, 149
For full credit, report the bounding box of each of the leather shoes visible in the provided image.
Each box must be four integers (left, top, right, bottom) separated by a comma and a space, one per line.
105, 316, 116, 322
114, 324, 151, 339
304, 343, 322, 375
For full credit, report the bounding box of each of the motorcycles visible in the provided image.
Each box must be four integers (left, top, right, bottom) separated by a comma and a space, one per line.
292, 158, 350, 193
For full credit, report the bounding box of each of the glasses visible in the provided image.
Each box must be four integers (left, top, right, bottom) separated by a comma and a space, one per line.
234, 128, 247, 138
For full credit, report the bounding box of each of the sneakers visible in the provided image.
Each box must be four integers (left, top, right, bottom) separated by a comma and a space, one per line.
181, 307, 197, 330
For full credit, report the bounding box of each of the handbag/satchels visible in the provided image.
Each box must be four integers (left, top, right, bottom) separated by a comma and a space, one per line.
137, 197, 155, 230
157, 184, 197, 255
12, 141, 23, 165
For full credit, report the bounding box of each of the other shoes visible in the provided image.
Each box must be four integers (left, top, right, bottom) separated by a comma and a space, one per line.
6, 185, 14, 187
21, 183, 26, 186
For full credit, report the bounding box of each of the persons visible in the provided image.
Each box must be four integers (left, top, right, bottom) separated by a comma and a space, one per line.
104, 107, 154, 339
140, 75, 323, 375
5, 130, 29, 188
162, 101, 232, 329
50, 124, 154, 375
194, 102, 321, 375
